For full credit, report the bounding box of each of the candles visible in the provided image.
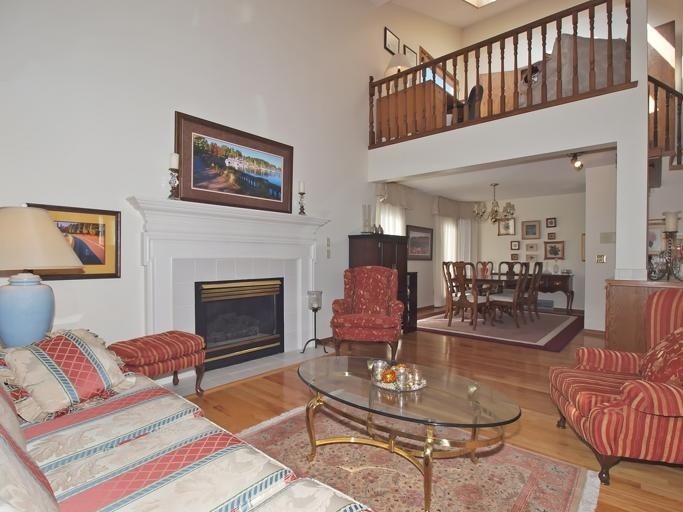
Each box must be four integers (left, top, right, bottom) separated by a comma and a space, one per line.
169, 153, 179, 169
299, 182, 306, 193
299, 291, 329, 353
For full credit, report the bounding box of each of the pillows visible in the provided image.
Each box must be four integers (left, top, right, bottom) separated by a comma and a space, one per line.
0, 329, 136, 425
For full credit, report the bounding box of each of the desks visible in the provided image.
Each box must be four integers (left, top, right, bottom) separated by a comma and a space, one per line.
377, 80, 457, 141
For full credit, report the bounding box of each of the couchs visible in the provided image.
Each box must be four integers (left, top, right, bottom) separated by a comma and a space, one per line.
330, 265, 404, 359
517, 34, 626, 108
0, 330, 375, 512
548, 287, 683, 485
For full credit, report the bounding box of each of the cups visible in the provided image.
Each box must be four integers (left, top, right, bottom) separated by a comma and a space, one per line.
371, 358, 423, 385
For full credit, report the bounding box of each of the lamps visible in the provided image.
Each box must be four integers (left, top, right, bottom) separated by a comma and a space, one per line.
646, 212, 682, 283
384, 54, 413, 92
0, 207, 87, 348
474, 182, 516, 225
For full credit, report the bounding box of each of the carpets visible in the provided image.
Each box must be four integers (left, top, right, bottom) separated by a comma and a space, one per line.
235, 387, 602, 512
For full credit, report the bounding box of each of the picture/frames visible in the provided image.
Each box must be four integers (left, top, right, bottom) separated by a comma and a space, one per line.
174, 110, 294, 213
525, 243, 537, 252
511, 241, 519, 250
406, 225, 433, 261
498, 218, 516, 236
384, 26, 400, 55
404, 44, 417, 66
521, 221, 540, 239
648, 219, 666, 255
25, 203, 121, 280
544, 241, 564, 260
511, 254, 518, 260
581, 233, 586, 261
526, 254, 536, 261
547, 232, 556, 240
546, 218, 556, 228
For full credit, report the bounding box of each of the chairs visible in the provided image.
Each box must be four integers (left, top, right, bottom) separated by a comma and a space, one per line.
489, 262, 527, 328
522, 262, 543, 320
442, 262, 474, 319
108, 331, 205, 397
447, 261, 491, 330
452, 85, 483, 124
453, 262, 469, 293
496, 261, 523, 292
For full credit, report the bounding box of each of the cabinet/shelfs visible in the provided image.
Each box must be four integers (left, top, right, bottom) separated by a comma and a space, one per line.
406, 272, 417, 332
349, 232, 408, 334
605, 279, 662, 352
